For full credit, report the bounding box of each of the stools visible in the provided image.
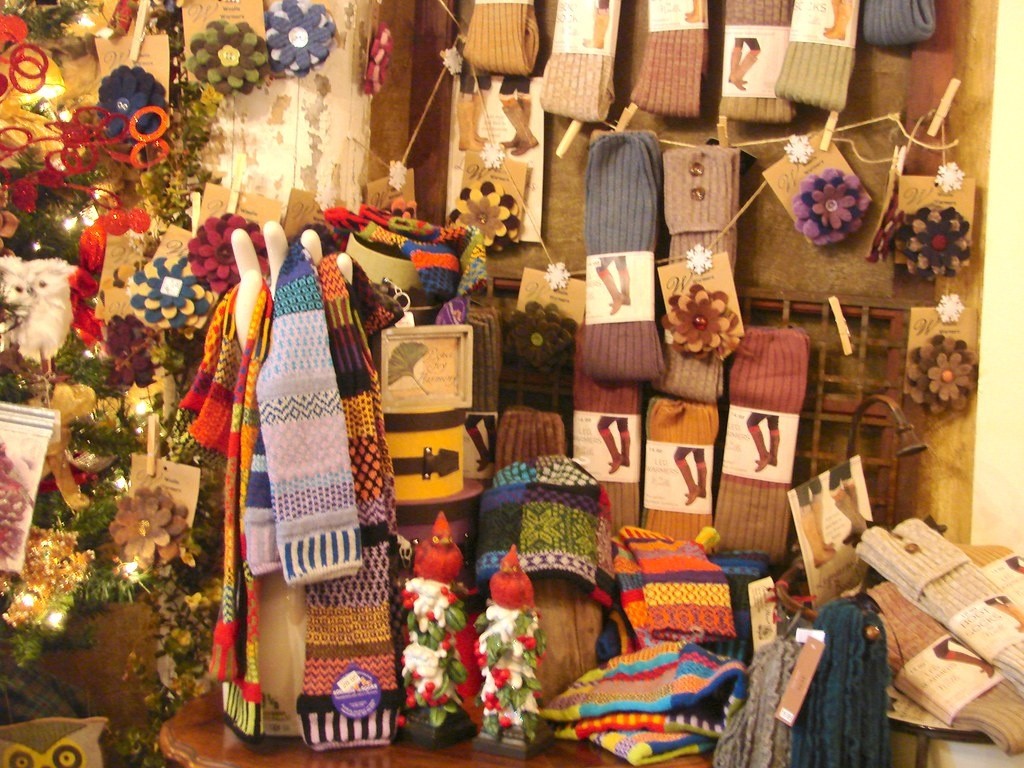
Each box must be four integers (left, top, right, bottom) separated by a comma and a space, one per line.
887, 683, 996, 768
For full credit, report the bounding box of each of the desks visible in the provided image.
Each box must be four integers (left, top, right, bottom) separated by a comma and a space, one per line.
159, 688, 713, 768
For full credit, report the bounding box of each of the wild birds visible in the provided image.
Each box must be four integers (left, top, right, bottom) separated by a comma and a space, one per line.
0, 253, 78, 378
